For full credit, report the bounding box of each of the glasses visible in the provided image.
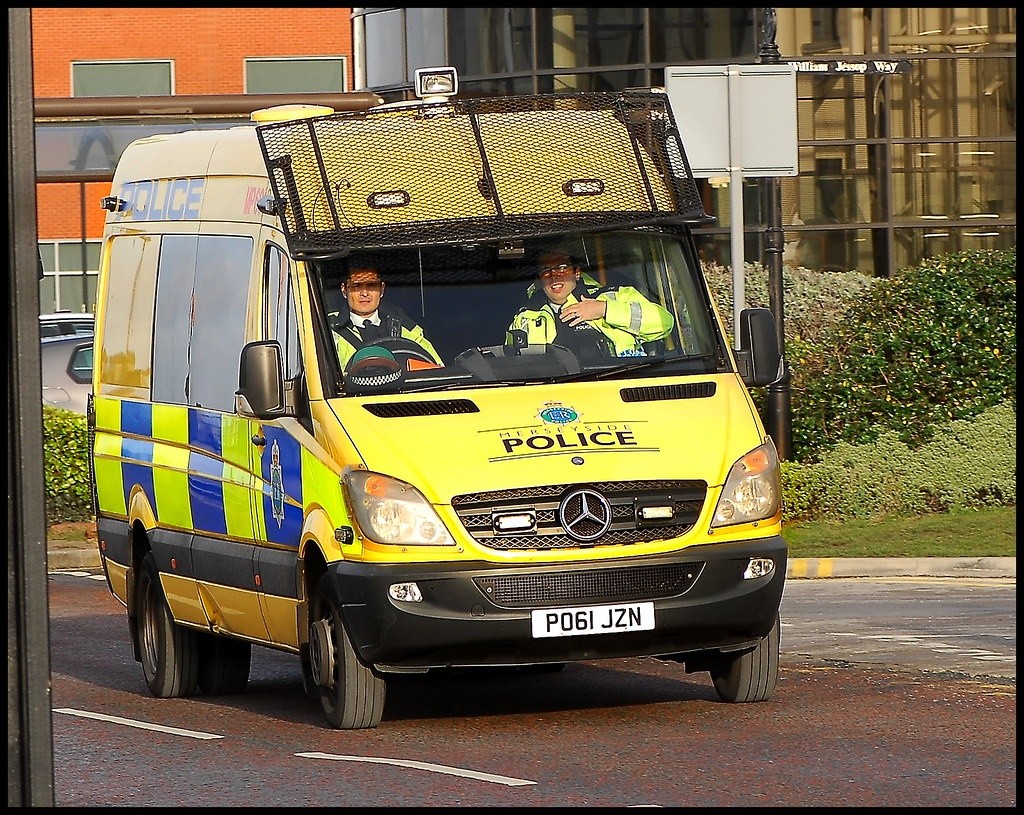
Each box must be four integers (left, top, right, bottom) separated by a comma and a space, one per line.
539, 263, 574, 276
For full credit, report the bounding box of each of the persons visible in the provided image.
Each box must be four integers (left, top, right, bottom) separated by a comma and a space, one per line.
318, 252, 446, 373
501, 243, 675, 361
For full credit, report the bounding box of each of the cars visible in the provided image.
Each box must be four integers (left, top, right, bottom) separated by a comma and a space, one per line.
36, 313, 94, 416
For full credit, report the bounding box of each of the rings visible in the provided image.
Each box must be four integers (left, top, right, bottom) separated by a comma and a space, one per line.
573, 312, 578, 318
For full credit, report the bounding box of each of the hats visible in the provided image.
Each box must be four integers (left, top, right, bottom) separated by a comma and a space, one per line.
345, 345, 405, 394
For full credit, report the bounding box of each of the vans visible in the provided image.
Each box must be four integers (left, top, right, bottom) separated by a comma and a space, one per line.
88, 68, 788, 730
